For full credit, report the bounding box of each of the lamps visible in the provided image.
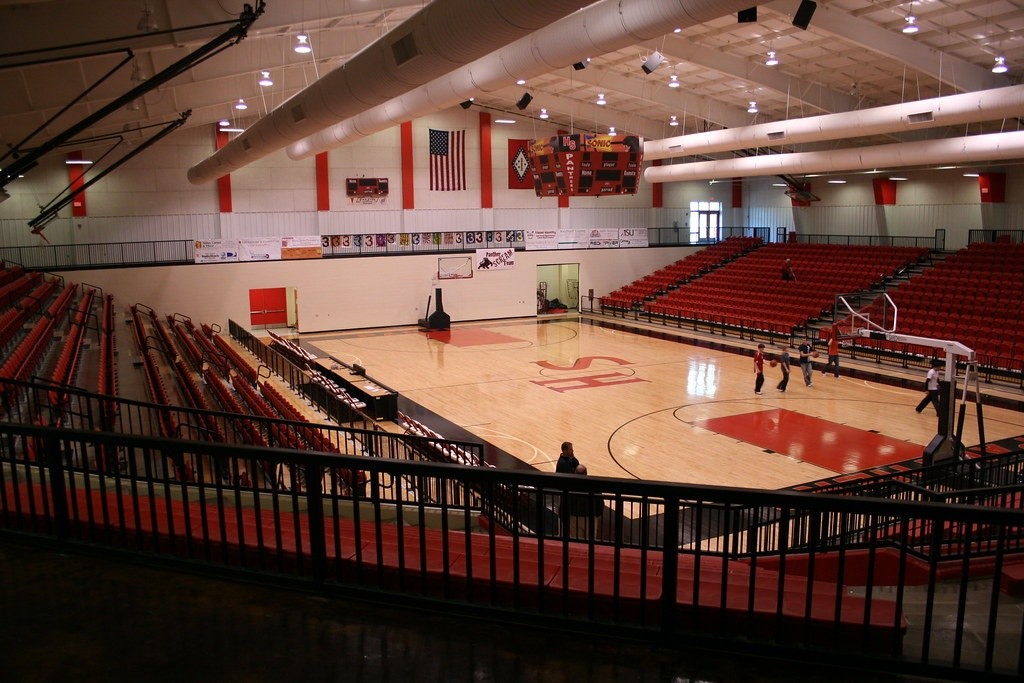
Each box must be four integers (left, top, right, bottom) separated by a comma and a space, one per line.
902, 0, 918, 33
125, 1, 159, 111
991, 40, 1007, 73
235, 98, 248, 110
259, 69, 273, 86
294, 0, 311, 52
540, 40, 779, 136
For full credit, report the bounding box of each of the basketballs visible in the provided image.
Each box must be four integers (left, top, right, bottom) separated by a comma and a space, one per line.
812, 350, 819, 359
770, 360, 778, 367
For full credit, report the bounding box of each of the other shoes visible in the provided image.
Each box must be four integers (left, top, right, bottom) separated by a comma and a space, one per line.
936, 412, 941, 417
833, 375, 840, 379
806, 383, 813, 387
915, 407, 921, 414
754, 390, 762, 394
820, 372, 826, 376
777, 388, 785, 392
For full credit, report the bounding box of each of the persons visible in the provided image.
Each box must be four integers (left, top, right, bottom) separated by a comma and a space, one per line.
821, 336, 842, 379
915, 361, 942, 417
753, 344, 771, 396
555, 442, 580, 475
566, 464, 604, 542
798, 336, 814, 387
782, 259, 794, 280
776, 346, 791, 392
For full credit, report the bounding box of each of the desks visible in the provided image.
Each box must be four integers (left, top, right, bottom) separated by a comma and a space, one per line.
349, 379, 399, 422
330, 367, 372, 393
314, 358, 350, 377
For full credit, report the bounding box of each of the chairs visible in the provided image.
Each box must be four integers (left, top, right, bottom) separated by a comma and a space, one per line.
0, 231, 1024, 683
